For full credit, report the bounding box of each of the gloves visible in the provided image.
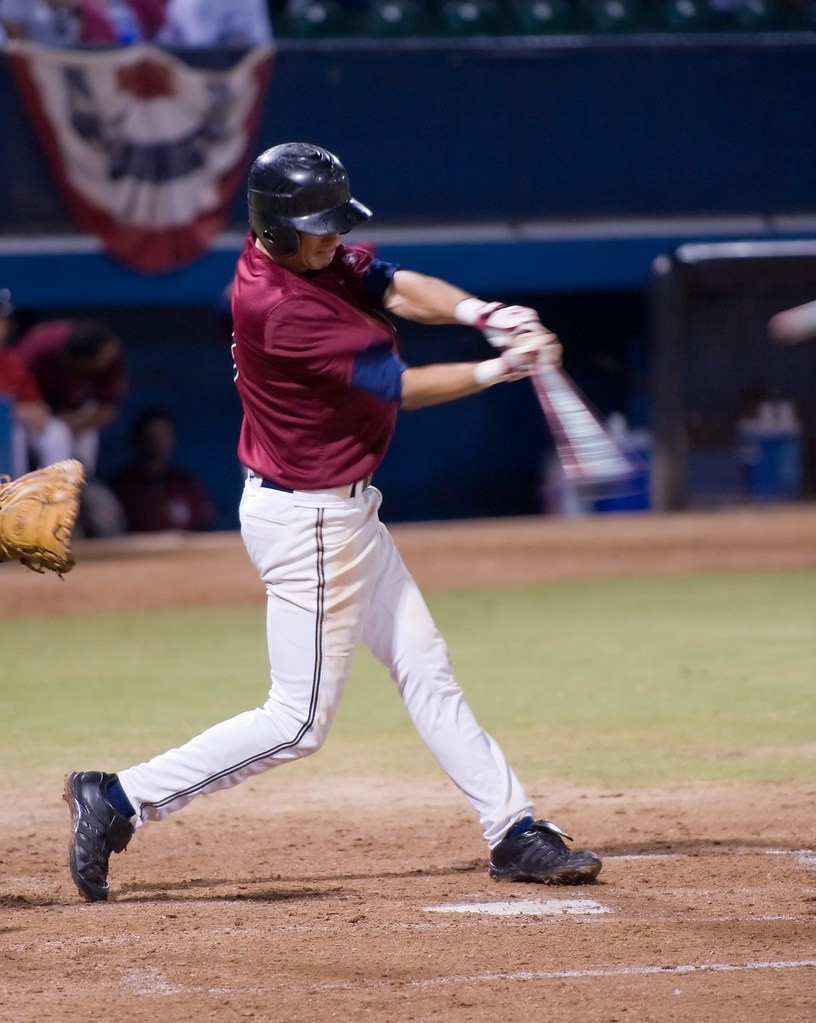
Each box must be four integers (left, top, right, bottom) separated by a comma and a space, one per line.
474, 301, 550, 349
498, 331, 562, 383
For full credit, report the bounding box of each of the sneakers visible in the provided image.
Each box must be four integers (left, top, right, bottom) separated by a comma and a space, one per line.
488, 819, 603, 886
63, 770, 135, 902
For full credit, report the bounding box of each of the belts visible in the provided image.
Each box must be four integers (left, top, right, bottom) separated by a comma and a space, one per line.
260, 474, 373, 498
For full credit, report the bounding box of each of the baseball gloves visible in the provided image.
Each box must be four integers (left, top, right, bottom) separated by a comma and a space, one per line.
0, 457, 88, 583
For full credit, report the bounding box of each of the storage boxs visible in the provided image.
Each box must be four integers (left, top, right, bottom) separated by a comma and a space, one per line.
544, 437, 651, 516
738, 422, 797, 504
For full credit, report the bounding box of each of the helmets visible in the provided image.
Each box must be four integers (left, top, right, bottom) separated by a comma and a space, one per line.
245, 143, 373, 258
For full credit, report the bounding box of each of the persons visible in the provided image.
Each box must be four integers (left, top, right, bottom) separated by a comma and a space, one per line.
60, 143, 604, 900
0, 285, 221, 540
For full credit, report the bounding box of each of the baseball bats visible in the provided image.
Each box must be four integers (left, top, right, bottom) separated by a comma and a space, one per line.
518, 336, 640, 486
